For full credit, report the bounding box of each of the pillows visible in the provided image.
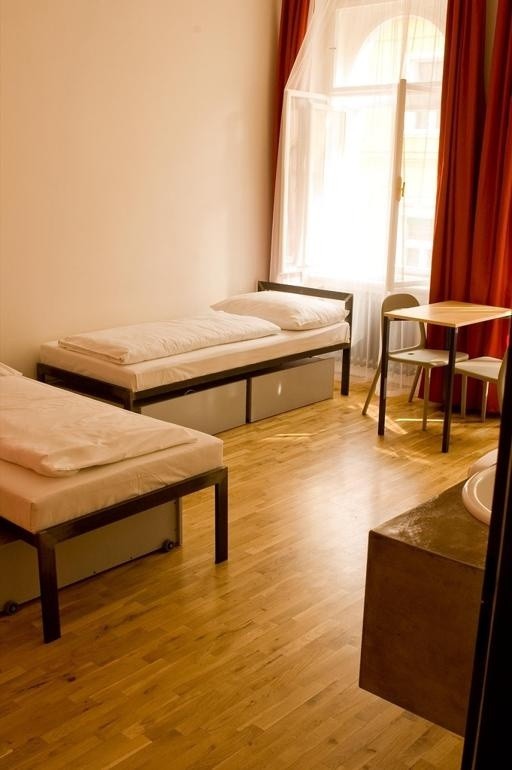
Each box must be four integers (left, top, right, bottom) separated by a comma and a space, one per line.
212, 290, 347, 330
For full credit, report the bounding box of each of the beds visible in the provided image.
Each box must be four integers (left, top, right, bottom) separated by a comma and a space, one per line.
39, 281, 353, 432
0, 356, 229, 647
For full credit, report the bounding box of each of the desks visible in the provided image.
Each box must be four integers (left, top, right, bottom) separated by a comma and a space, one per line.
377, 300, 512, 451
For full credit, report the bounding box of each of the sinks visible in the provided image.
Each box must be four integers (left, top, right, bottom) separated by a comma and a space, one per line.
462, 463, 498, 525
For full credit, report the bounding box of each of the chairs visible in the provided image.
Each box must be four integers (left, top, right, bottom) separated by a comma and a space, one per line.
361, 293, 512, 430
